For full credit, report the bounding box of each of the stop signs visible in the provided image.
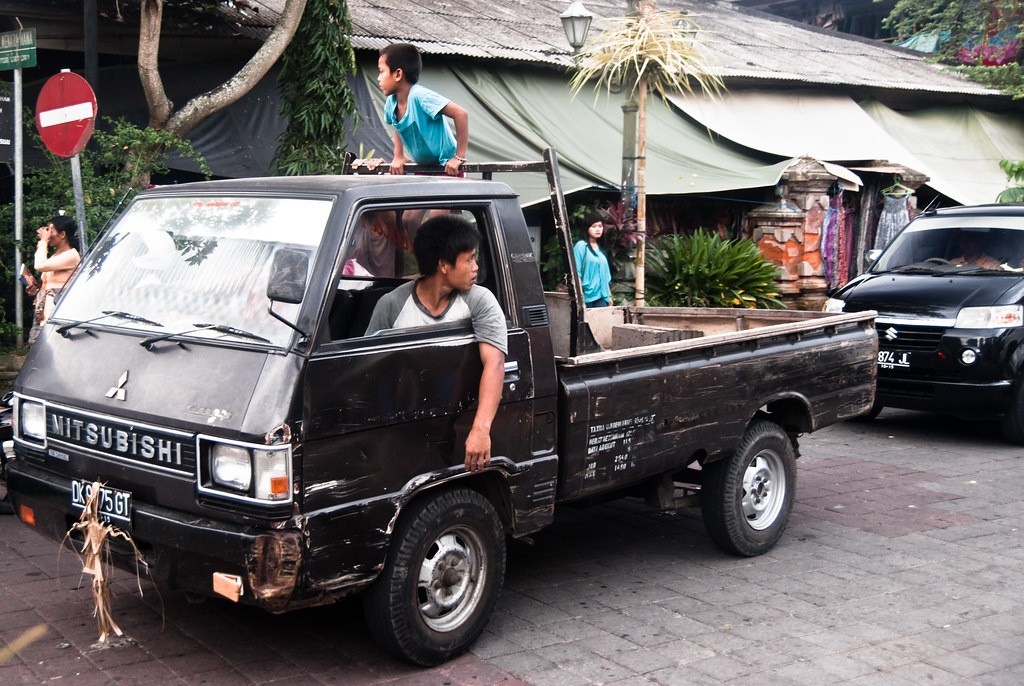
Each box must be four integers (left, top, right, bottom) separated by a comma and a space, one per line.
35, 71, 97, 159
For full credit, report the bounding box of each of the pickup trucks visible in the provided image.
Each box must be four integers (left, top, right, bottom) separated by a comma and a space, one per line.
818, 193, 1024, 445
7, 145, 883, 668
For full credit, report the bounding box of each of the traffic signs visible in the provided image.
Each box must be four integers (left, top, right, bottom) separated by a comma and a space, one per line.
0, 28, 36, 73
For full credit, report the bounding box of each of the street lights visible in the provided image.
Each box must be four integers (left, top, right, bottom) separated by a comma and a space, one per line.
564, 0, 698, 226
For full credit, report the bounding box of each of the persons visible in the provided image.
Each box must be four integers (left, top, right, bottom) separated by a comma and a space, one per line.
377, 41, 471, 250
360, 215, 508, 474
572, 211, 614, 309
352, 210, 396, 276
27, 216, 81, 346
944, 230, 1003, 271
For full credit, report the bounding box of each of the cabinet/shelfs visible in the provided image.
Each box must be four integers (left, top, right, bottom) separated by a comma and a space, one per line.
880, 174, 916, 195
